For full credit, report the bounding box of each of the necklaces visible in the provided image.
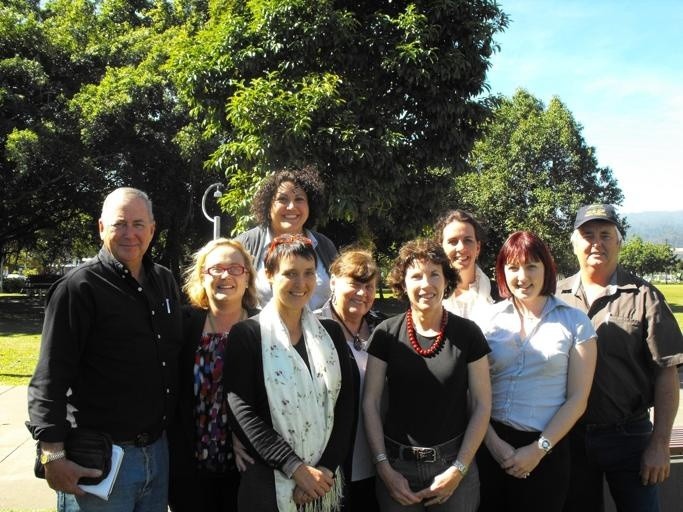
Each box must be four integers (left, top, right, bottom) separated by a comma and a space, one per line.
403, 308, 449, 357
207, 304, 244, 334
329, 303, 364, 352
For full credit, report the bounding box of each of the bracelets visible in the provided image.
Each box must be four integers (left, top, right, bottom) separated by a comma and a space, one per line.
371, 453, 388, 465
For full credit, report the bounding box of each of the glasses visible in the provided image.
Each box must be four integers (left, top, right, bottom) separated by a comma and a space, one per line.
267, 235, 312, 263
207, 266, 244, 275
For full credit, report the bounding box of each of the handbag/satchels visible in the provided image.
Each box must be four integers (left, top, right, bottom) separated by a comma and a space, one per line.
36, 427, 112, 484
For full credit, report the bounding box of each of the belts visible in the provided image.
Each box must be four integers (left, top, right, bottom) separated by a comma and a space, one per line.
383, 435, 463, 462
118, 426, 164, 448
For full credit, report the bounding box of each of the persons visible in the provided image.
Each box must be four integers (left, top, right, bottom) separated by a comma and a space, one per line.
231, 165, 340, 311
25, 187, 182, 512
481, 230, 598, 512
223, 232, 360, 512
431, 209, 505, 320
312, 245, 390, 512
168, 238, 262, 512
554, 204, 683, 512
362, 236, 492, 512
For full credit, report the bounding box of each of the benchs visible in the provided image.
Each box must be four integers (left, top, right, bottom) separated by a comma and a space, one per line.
20, 274, 64, 295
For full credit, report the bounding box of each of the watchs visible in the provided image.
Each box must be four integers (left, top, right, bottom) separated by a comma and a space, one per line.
452, 459, 469, 476
536, 436, 552, 455
37, 450, 66, 465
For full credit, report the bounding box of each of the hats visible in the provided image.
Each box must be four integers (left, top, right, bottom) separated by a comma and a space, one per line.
574, 204, 618, 229
